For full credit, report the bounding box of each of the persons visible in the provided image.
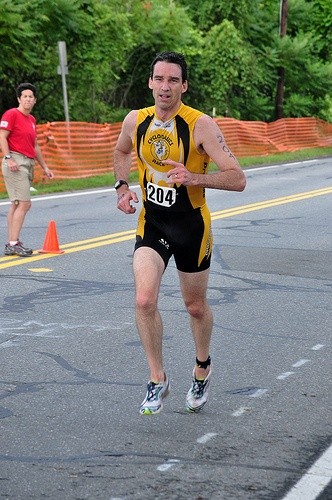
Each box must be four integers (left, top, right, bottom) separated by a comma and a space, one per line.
0, 82, 54, 257
113, 50, 247, 416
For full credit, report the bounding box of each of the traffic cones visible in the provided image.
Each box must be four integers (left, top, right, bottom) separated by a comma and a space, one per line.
38, 219, 65, 255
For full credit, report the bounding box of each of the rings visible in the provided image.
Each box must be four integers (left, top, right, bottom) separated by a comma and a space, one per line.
175, 175, 178, 178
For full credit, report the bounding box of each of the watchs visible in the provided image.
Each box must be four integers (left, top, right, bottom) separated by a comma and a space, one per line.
114, 179, 129, 190
5, 154, 11, 159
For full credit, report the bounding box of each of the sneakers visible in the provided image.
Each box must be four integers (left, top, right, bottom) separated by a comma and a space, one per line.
139, 372, 170, 414
185, 364, 212, 413
4, 240, 33, 257
